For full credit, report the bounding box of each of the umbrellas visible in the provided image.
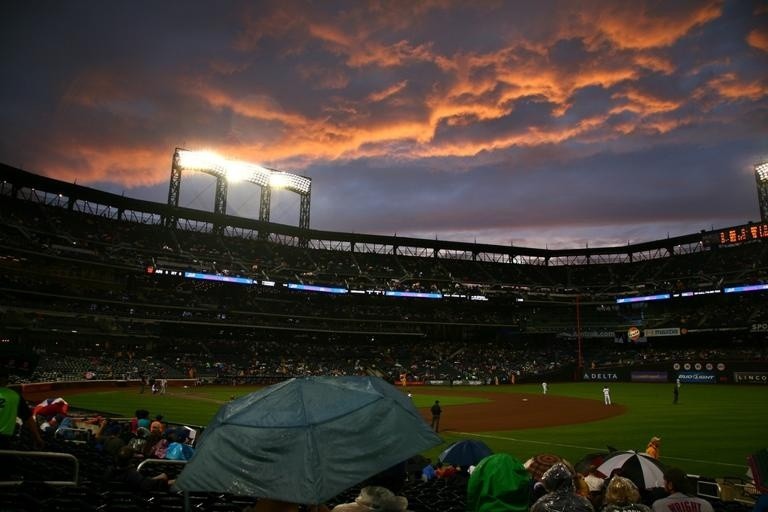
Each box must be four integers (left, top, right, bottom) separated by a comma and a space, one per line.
438, 438, 496, 466
174, 373, 451, 507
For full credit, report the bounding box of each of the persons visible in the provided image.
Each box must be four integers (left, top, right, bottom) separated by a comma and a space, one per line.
672, 382, 679, 403
0, 394, 207, 511
407, 391, 412, 398
601, 385, 611, 405
248, 448, 767, 511
646, 437, 663, 459
1, 201, 767, 395
430, 400, 443, 433
